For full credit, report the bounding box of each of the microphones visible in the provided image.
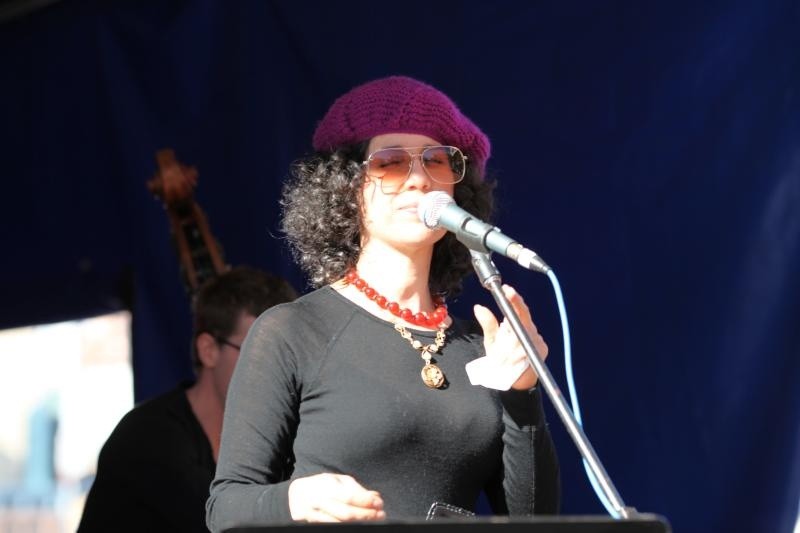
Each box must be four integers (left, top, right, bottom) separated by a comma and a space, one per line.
416, 190, 552, 273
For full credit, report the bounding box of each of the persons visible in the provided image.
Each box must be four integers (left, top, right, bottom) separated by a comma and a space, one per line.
205, 76, 562, 533
74, 269, 298, 533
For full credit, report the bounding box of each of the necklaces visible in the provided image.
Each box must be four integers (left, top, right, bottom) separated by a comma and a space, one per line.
348, 269, 449, 326
387, 315, 453, 389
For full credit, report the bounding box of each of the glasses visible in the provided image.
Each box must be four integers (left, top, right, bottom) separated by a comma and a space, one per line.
357, 143, 468, 187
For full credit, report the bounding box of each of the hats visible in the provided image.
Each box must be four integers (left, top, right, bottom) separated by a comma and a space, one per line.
308, 74, 491, 181
